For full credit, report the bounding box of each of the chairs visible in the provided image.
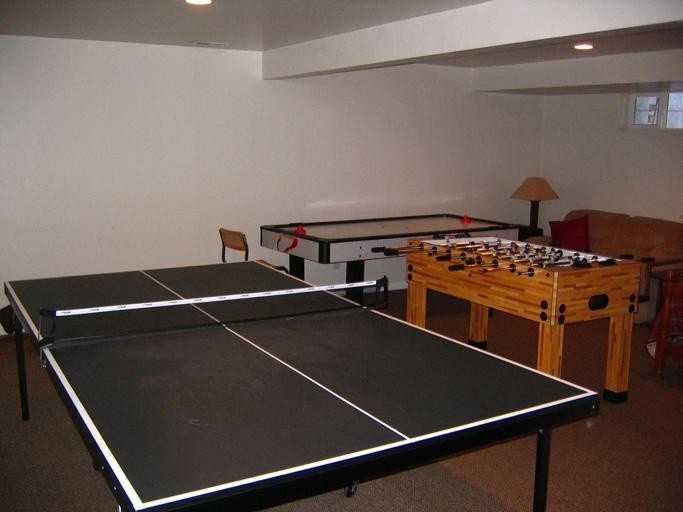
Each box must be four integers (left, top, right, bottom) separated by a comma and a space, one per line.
217, 227, 291, 279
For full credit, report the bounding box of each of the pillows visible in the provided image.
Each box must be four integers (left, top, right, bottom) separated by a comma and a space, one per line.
548, 214, 591, 252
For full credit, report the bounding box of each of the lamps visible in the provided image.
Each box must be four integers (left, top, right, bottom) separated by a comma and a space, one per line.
508, 177, 560, 236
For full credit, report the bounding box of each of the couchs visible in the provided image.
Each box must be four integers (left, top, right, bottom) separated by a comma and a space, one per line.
522, 206, 683, 327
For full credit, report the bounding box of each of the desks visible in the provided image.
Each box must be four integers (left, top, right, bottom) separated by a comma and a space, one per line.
260, 212, 520, 303
2, 259, 600, 512
